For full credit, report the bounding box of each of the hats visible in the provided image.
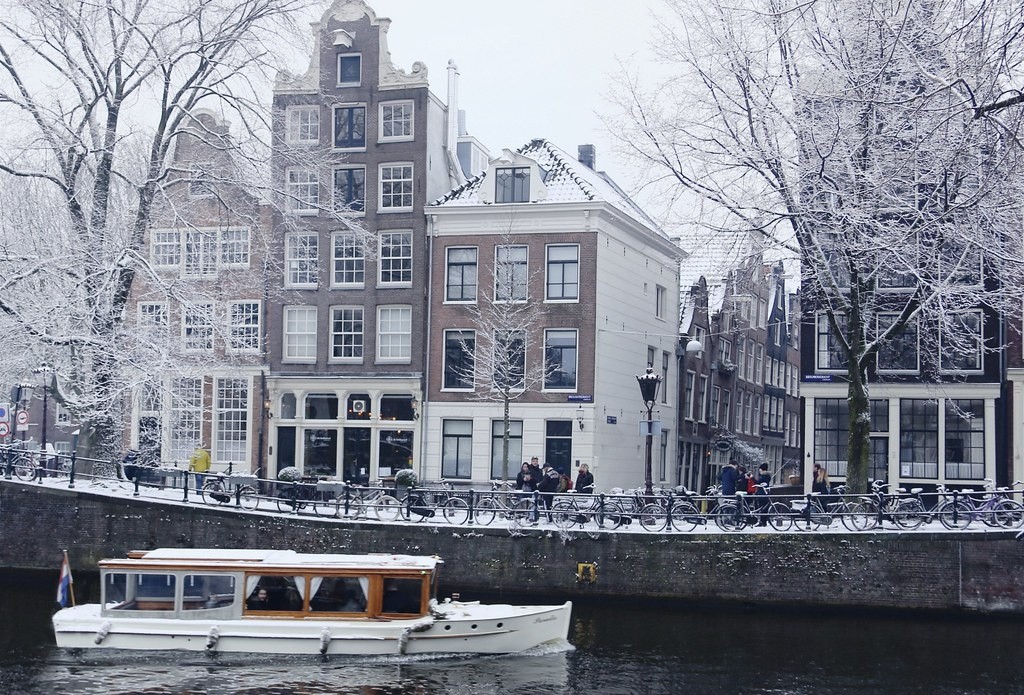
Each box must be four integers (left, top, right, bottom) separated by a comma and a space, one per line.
532, 456, 539, 460
556, 467, 564, 474
729, 460, 738, 465
542, 463, 551, 469
759, 462, 768, 471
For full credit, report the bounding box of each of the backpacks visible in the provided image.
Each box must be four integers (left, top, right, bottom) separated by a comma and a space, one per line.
747, 475, 756, 493
563, 475, 573, 490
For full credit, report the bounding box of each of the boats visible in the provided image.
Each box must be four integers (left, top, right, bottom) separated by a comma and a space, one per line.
50, 545, 575, 661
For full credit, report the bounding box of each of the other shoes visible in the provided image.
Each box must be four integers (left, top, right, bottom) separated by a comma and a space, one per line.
755, 519, 767, 527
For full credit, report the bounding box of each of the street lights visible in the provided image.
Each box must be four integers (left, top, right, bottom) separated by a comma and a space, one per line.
634, 362, 666, 525
17, 382, 34, 448
30, 361, 58, 478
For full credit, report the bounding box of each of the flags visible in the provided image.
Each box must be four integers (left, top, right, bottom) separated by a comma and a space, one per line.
57, 557, 74, 607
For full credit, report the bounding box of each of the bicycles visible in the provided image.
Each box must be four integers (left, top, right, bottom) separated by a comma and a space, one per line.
276, 476, 340, 519
201, 468, 261, 511
0, 437, 38, 482
401, 477, 469, 527
474, 477, 542, 527
335, 480, 402, 522
551, 483, 1024, 534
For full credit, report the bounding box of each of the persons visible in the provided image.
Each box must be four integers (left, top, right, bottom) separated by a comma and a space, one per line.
244, 582, 411, 613
717, 457, 831, 527
189, 444, 211, 494
45, 439, 56, 477
517, 456, 593, 522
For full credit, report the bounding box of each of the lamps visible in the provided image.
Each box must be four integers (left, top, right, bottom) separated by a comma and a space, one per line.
576, 402, 586, 430
263, 396, 273, 419
